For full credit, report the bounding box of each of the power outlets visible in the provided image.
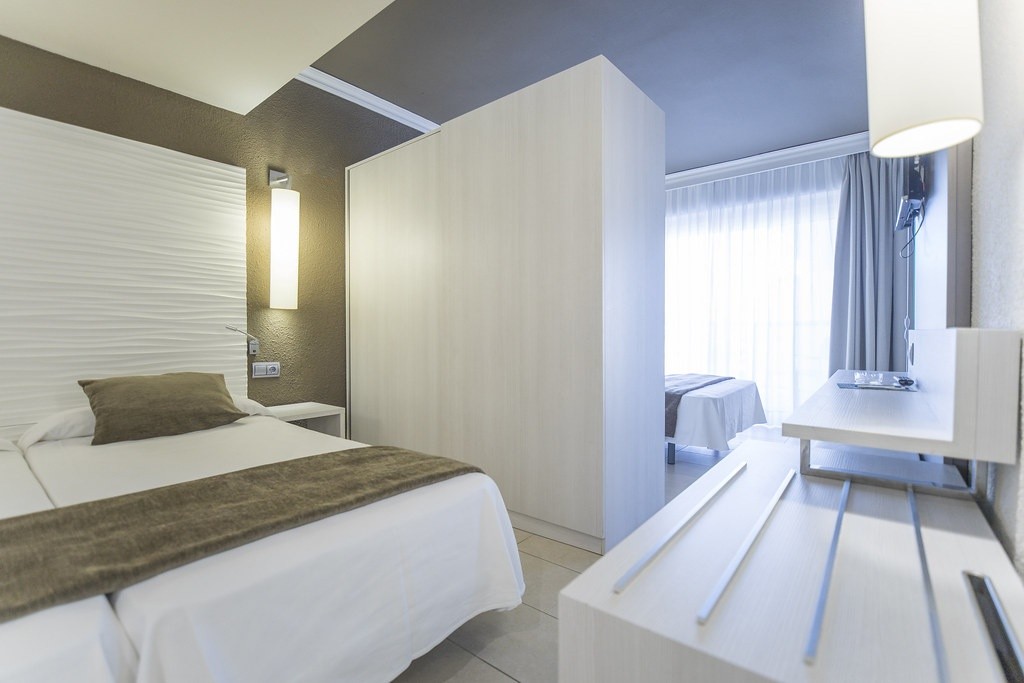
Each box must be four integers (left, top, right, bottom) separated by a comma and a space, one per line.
252, 362, 281, 378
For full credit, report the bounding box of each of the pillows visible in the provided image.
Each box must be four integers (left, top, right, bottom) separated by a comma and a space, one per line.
18, 394, 279, 455
78, 371, 250, 445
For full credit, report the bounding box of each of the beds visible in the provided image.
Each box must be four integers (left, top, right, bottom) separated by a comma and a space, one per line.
664, 372, 766, 465
0, 409, 526, 683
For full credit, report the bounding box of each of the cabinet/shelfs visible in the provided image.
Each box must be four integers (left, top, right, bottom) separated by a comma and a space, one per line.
558, 327, 1024, 683
344, 55, 669, 560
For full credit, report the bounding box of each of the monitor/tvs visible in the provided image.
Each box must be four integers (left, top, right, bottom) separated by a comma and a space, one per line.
895, 156, 924, 231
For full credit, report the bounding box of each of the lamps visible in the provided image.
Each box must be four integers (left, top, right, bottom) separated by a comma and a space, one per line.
863, 0, 983, 158
265, 170, 301, 309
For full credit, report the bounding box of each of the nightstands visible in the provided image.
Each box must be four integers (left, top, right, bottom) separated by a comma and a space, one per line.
268, 402, 346, 439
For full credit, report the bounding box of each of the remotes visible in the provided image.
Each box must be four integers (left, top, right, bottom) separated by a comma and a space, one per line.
894, 376, 914, 385
